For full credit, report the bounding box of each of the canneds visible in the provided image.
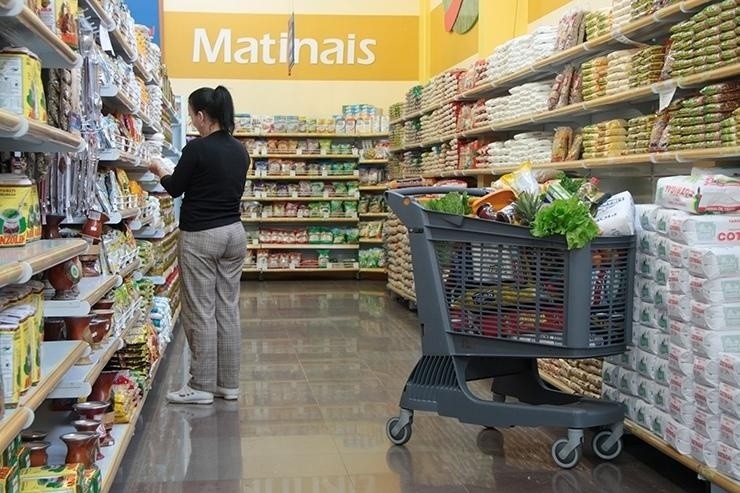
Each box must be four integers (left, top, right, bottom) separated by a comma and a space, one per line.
476, 200, 518, 225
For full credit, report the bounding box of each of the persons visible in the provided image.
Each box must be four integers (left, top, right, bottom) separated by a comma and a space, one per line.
147, 85, 250, 405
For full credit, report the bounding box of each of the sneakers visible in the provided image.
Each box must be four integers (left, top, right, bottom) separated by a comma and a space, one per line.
215, 383, 240, 401
166, 384, 214, 406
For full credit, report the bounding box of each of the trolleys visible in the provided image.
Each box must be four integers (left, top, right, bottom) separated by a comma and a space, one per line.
384, 184, 629, 469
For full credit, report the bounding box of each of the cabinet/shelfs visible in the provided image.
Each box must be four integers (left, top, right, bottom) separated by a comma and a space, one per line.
0, 0, 185, 493
387, 2, 739, 492
186, 131, 387, 280
